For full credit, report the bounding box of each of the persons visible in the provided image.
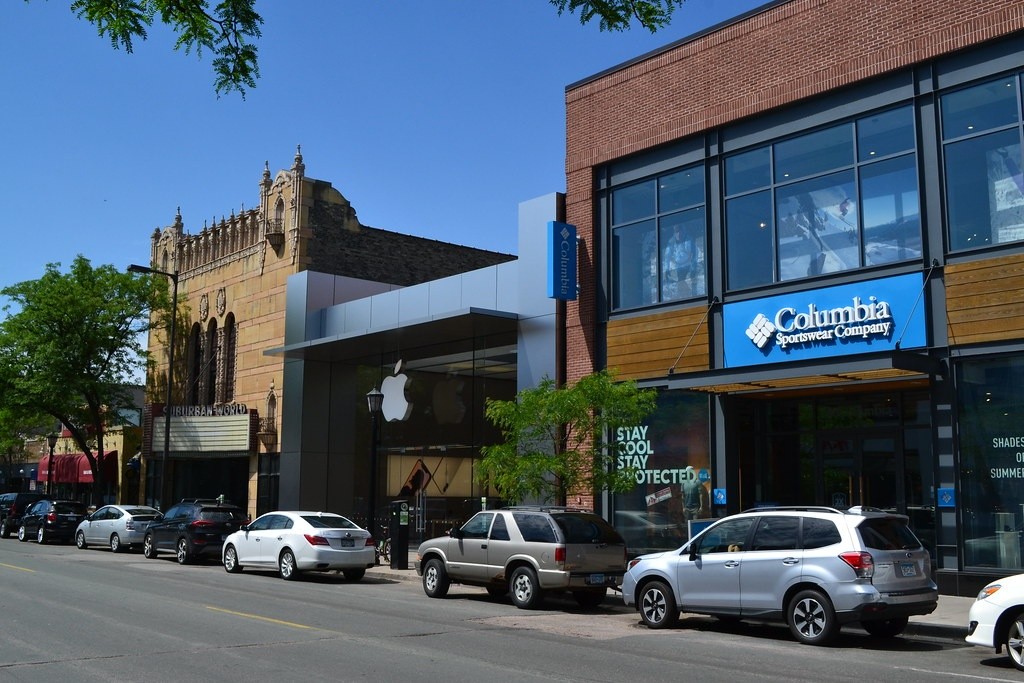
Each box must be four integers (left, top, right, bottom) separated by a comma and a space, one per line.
681, 466, 703, 527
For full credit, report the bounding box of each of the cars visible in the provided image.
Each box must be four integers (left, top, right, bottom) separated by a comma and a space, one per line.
965, 574, 1023, 671
614, 511, 682, 557
74, 504, 165, 553
221, 510, 376, 581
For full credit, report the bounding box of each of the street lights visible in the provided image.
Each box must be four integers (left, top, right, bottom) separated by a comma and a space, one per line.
366, 384, 386, 547
45, 432, 59, 495
126, 262, 179, 502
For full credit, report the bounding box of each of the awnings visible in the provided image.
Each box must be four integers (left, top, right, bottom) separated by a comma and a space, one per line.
38, 450, 118, 483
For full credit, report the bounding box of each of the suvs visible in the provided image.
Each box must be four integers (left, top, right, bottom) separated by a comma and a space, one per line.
414, 505, 628, 610
621, 504, 938, 646
17, 499, 88, 544
0, 492, 53, 538
143, 498, 252, 565
